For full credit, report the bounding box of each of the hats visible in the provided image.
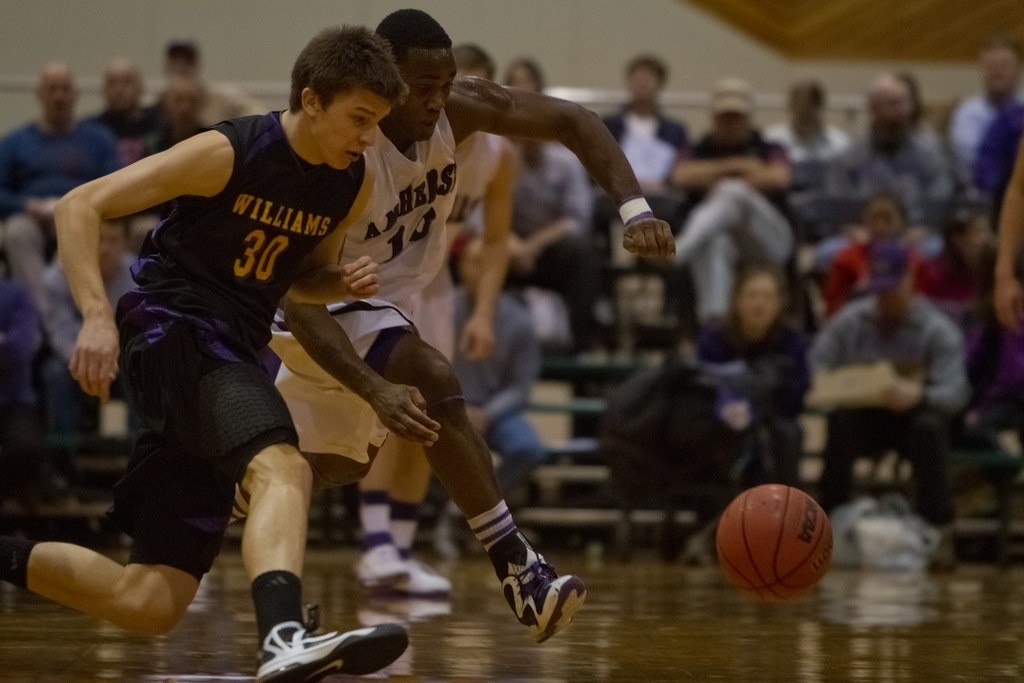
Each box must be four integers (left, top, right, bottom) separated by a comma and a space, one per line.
866, 241, 907, 293
712, 79, 753, 115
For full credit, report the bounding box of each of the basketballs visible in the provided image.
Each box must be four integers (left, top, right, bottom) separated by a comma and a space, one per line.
715, 483, 834, 606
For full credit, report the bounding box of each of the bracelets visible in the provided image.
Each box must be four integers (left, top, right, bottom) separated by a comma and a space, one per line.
619, 196, 654, 226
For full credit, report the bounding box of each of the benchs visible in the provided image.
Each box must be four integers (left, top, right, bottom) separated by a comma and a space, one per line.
0, 333, 1024, 563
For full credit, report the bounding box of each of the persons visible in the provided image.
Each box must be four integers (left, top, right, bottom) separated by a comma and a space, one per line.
273, 8, 591, 644
0, 37, 1021, 591
0, 25, 408, 683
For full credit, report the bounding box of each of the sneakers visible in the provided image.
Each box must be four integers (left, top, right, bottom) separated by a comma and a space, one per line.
501, 547, 586, 644
358, 544, 410, 588
256, 606, 409, 683
391, 556, 451, 593
